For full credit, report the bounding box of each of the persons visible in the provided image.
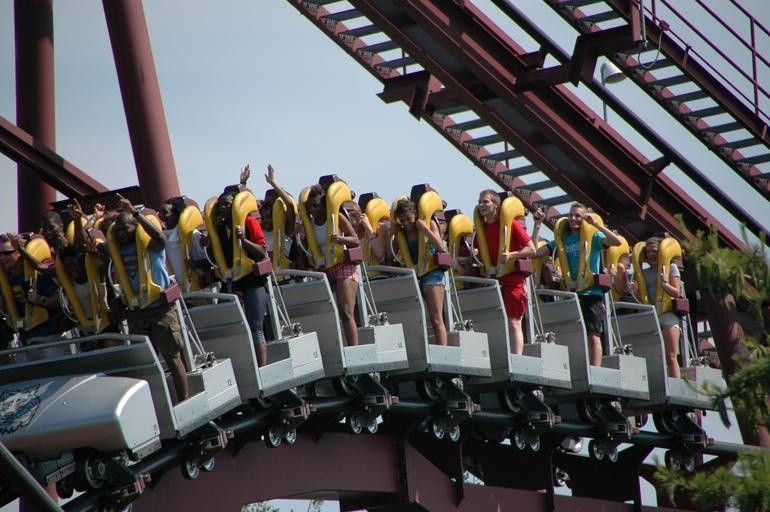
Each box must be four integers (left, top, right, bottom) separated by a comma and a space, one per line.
451, 189, 682, 380
0, 163, 448, 402
